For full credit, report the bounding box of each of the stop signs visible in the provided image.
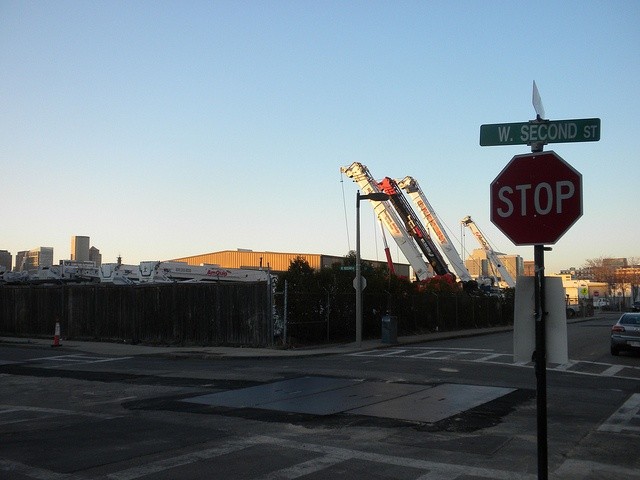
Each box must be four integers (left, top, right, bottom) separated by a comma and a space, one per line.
490, 150, 583, 246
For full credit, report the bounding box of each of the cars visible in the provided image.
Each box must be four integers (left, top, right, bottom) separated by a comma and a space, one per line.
594, 297, 610, 307
611, 312, 640, 355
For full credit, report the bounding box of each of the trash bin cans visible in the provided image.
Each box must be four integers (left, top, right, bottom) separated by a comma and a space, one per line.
381, 315, 399, 344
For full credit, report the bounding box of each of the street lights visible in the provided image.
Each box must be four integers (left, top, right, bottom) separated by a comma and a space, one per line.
356, 189, 389, 348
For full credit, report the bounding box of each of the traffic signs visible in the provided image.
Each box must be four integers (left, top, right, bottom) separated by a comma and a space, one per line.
479, 118, 600, 147
